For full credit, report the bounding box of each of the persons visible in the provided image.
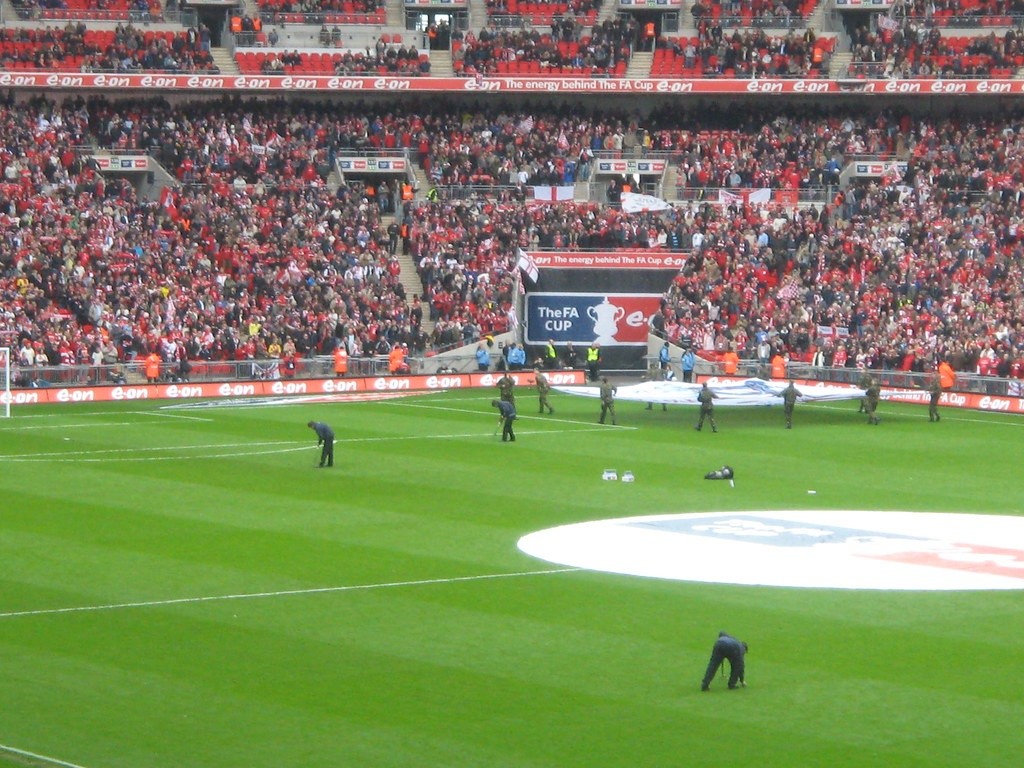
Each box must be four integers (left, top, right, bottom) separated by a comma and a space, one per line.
308, 421, 334, 469
532, 367, 555, 415
492, 401, 516, 442
644, 362, 667, 411
695, 383, 720, 433
0, 0, 1024, 388
681, 345, 696, 383
664, 365, 676, 381
701, 633, 748, 692
597, 377, 617, 425
929, 376, 942, 421
779, 380, 802, 429
866, 379, 881, 426
585, 343, 602, 382
857, 370, 872, 412
496, 372, 519, 420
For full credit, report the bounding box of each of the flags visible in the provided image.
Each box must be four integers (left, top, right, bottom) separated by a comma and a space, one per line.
512, 265, 526, 295
518, 249, 539, 284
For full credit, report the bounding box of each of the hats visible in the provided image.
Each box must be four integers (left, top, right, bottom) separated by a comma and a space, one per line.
402, 342, 407, 347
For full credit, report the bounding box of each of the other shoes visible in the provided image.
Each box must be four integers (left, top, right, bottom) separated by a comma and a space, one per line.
702, 685, 710, 690
694, 425, 700, 431
598, 421, 604, 424
499, 438, 508, 442
645, 407, 652, 410
728, 682, 739, 689
314, 463, 324, 469
713, 430, 717, 432
875, 416, 880, 425
663, 407, 667, 411
612, 421, 616, 424
935, 415, 940, 421
508, 436, 515, 442
538, 411, 544, 413
928, 420, 934, 422
787, 426, 792, 429
324, 463, 334, 467
549, 407, 554, 414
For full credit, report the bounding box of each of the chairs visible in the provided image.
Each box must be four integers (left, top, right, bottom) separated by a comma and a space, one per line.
0, 0, 1024, 79
